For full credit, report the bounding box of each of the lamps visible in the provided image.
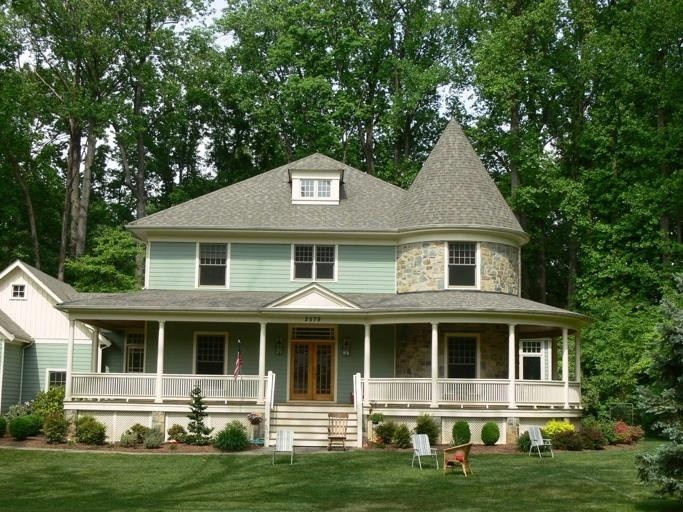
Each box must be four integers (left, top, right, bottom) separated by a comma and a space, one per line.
276, 339, 283, 355
342, 340, 350, 357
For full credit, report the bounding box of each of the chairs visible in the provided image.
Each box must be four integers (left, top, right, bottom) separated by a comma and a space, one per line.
527, 426, 554, 459
411, 434, 474, 477
272, 428, 294, 465
327, 411, 349, 451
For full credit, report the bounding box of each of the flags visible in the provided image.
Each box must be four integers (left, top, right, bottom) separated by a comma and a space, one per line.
232, 351, 243, 380
